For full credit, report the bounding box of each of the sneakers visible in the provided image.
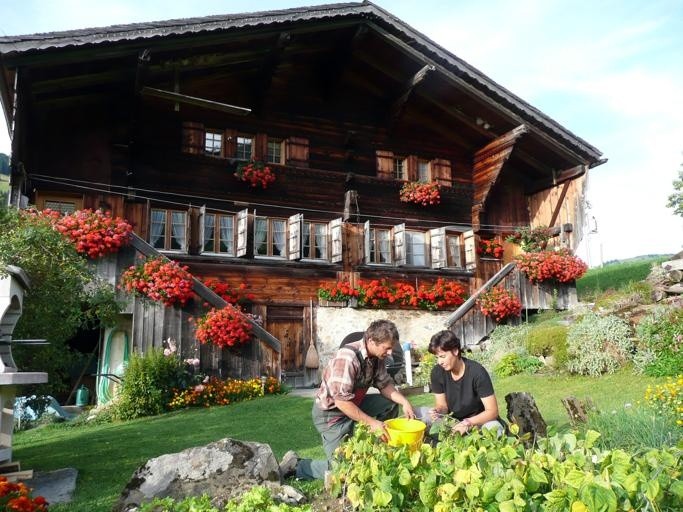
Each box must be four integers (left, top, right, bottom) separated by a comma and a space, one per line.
280, 450, 300, 480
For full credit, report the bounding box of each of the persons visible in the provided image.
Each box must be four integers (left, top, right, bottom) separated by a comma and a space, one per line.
413, 330, 507, 450
280, 320, 416, 482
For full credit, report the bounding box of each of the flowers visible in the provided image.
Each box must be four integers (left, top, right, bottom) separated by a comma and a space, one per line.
50, 207, 256, 349
317, 224, 591, 322
235, 158, 278, 190
398, 178, 444, 208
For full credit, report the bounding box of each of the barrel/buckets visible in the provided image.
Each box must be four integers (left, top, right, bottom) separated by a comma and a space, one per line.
381, 417, 427, 451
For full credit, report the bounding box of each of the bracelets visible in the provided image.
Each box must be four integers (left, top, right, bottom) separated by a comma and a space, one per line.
463, 417, 474, 427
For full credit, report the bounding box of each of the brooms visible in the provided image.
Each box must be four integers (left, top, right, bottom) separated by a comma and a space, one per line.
304, 297, 319, 368
